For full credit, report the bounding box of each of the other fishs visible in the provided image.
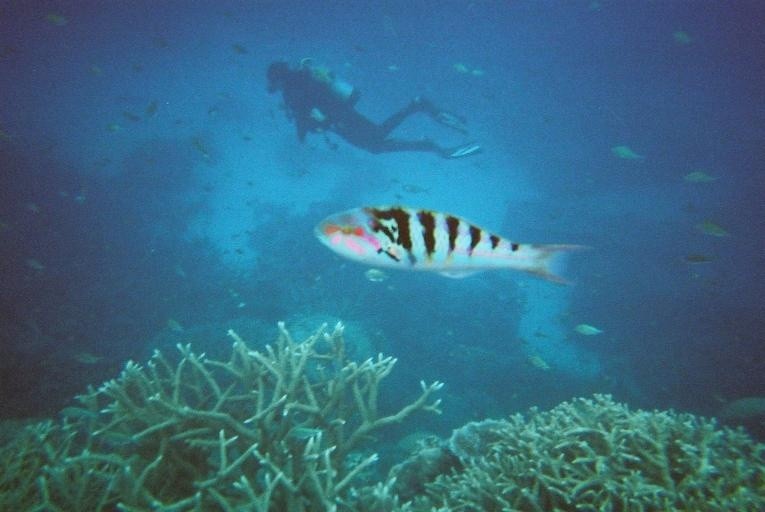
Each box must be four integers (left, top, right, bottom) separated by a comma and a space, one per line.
0, 11, 765, 456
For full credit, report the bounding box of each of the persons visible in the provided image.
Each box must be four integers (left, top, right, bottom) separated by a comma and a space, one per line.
266, 59, 451, 158
